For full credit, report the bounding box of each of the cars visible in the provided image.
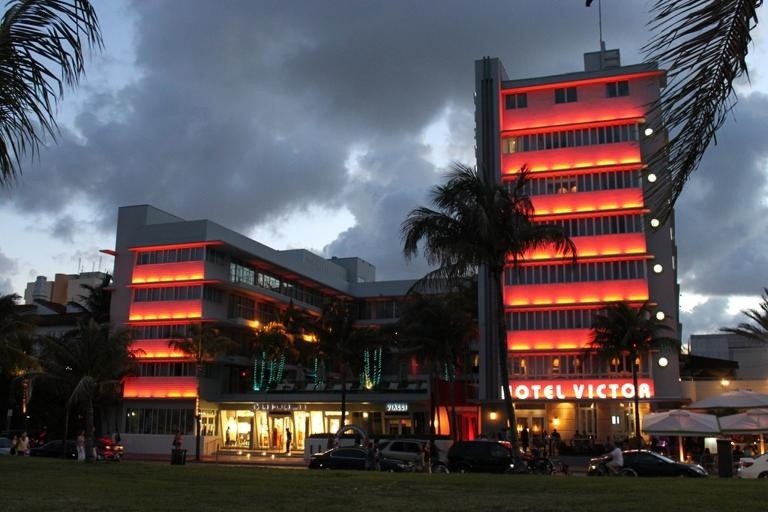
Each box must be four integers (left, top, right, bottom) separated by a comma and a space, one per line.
734, 450, 768, 481
0, 436, 126, 462
298, 430, 528, 477
585, 448, 708, 478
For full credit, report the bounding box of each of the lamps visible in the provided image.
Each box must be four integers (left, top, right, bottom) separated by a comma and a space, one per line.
553, 415, 560, 429
363, 411, 368, 420
487, 402, 497, 422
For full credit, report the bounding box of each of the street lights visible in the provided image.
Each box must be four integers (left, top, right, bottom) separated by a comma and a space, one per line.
190, 407, 201, 462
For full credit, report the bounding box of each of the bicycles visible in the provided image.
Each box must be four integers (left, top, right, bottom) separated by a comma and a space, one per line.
589, 456, 638, 477
503, 455, 553, 475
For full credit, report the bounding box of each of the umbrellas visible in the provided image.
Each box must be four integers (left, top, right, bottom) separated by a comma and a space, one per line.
643, 390, 768, 437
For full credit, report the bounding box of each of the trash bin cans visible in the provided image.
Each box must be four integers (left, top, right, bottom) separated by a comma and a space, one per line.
171, 449, 187, 465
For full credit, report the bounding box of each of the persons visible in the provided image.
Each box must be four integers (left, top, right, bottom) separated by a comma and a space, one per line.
424, 438, 445, 464
572, 429, 700, 477
284, 428, 292, 457
701, 443, 760, 470
225, 425, 232, 446
76, 428, 122, 461
172, 429, 181, 448
8, 426, 52, 455
520, 426, 561, 458
